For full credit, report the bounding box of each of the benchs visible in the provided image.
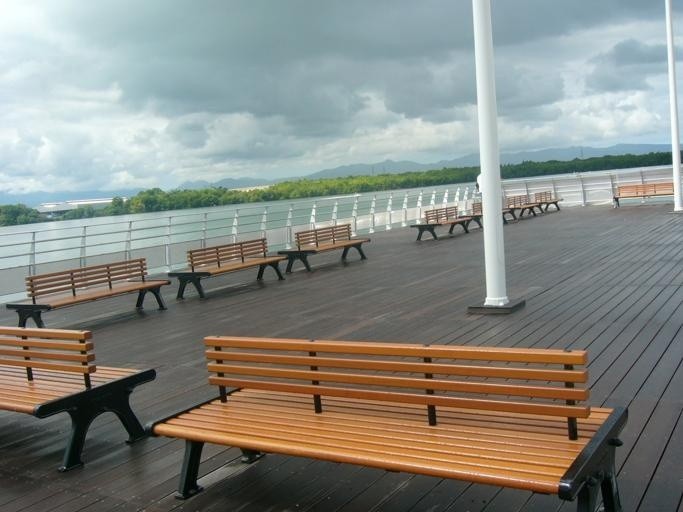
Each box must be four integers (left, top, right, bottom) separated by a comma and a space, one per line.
611, 180, 674, 209
5, 257, 171, 339
168, 237, 289, 303
277, 223, 371, 274
0, 326, 165, 476
143, 333, 631, 511
410, 189, 562, 242
